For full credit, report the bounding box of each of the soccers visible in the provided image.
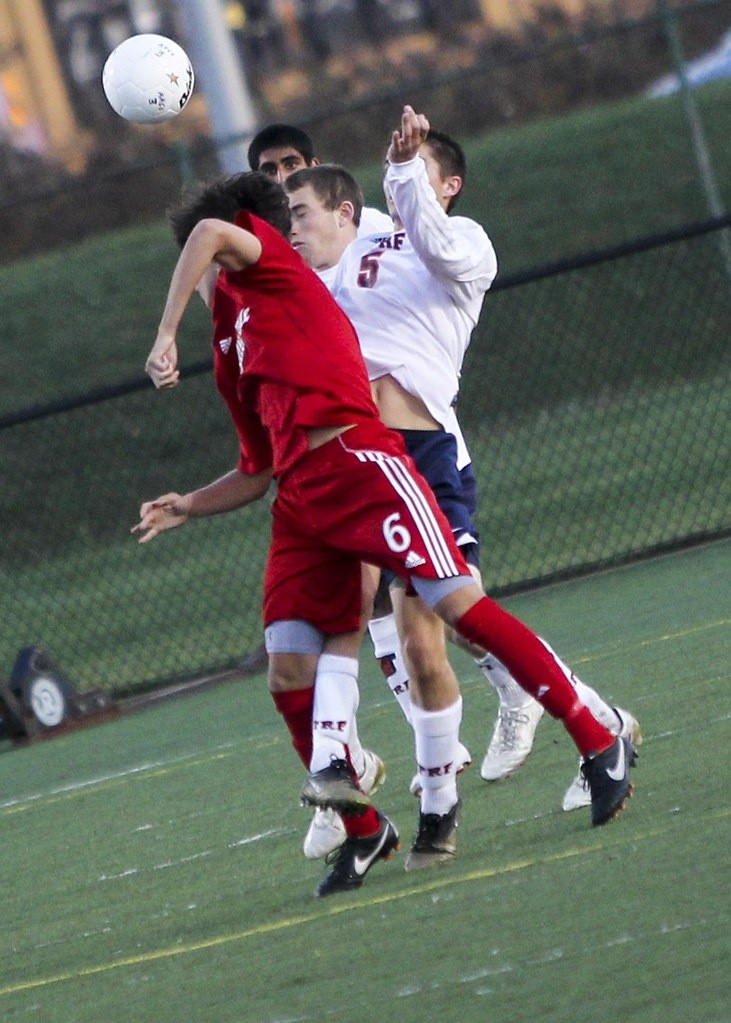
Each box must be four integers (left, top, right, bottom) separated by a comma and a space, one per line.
102, 33, 195, 125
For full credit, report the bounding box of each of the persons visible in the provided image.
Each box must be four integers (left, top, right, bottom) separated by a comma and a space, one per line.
128, 170, 643, 897
244, 101, 593, 859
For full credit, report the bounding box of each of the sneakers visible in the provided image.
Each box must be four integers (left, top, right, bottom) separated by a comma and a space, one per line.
302, 749, 386, 858
580, 736, 643, 827
480, 695, 544, 780
404, 798, 463, 872
313, 813, 403, 898
299, 753, 371, 819
562, 706, 639, 811
409, 742, 471, 798
